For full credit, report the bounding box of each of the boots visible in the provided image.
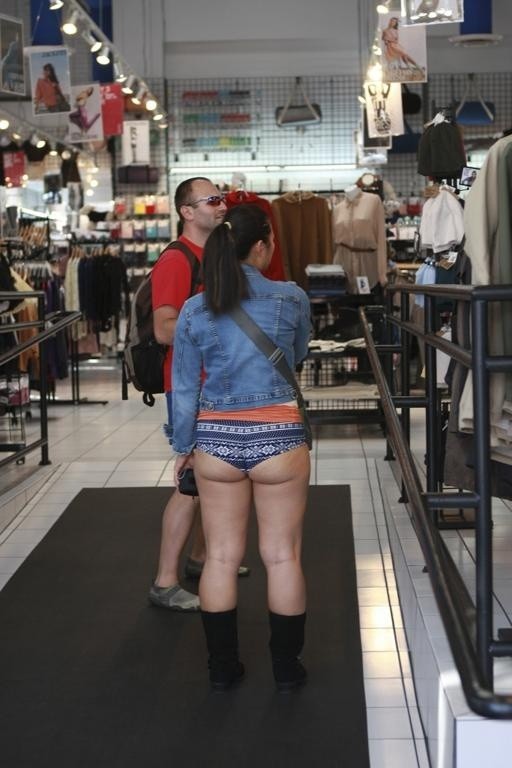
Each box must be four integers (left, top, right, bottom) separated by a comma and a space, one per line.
265, 609, 310, 695
202, 609, 245, 693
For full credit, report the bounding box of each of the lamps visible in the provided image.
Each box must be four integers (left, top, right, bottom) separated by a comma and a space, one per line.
114, 56, 128, 82
144, 93, 158, 111
49, 137, 58, 156
11, 125, 29, 143
97, 45, 110, 65
61, 10, 79, 35
49, 1, 64, 11
270, 164, 358, 169
170, 166, 268, 173
132, 87, 146, 105
32, 131, 44, 148
122, 73, 135, 94
81, 25, 103, 53
153, 107, 163, 122
158, 119, 168, 129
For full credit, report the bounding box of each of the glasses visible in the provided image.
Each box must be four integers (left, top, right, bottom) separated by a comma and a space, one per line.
189, 194, 229, 207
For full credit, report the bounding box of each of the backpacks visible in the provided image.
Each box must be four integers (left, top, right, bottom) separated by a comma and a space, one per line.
123, 240, 200, 405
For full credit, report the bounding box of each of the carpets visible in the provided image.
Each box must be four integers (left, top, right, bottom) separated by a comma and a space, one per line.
0, 484, 366, 765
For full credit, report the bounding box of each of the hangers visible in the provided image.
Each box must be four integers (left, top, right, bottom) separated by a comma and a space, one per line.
294, 183, 308, 203
17, 216, 51, 245
424, 108, 450, 127
12, 261, 54, 285
232, 183, 249, 199
68, 242, 114, 257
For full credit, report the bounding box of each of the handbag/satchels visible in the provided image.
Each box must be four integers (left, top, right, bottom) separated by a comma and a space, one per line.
389, 120, 419, 153
455, 75, 494, 125
275, 77, 322, 126
401, 83, 420, 114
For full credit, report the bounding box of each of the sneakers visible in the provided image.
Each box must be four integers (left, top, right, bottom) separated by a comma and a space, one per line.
148, 582, 200, 614
184, 559, 249, 580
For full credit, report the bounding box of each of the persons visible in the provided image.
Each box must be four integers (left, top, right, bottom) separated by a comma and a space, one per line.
35, 63, 63, 112
1, 31, 22, 90
68, 86, 101, 133
148, 177, 252, 610
332, 185, 389, 299
383, 18, 420, 70
169, 203, 312, 695
